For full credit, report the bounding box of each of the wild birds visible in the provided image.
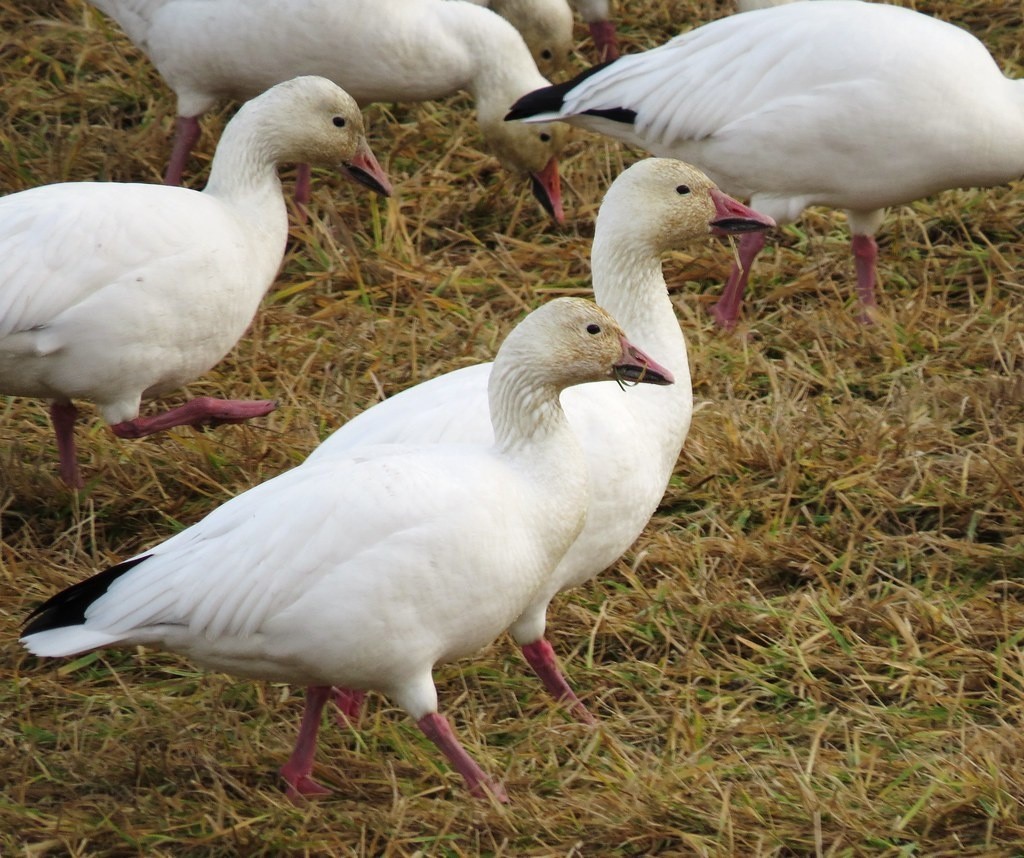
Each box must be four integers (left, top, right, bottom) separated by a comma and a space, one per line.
10, 157, 777, 809
0, 75, 393, 502
88, 0, 619, 234
504, 0, 1023, 340
